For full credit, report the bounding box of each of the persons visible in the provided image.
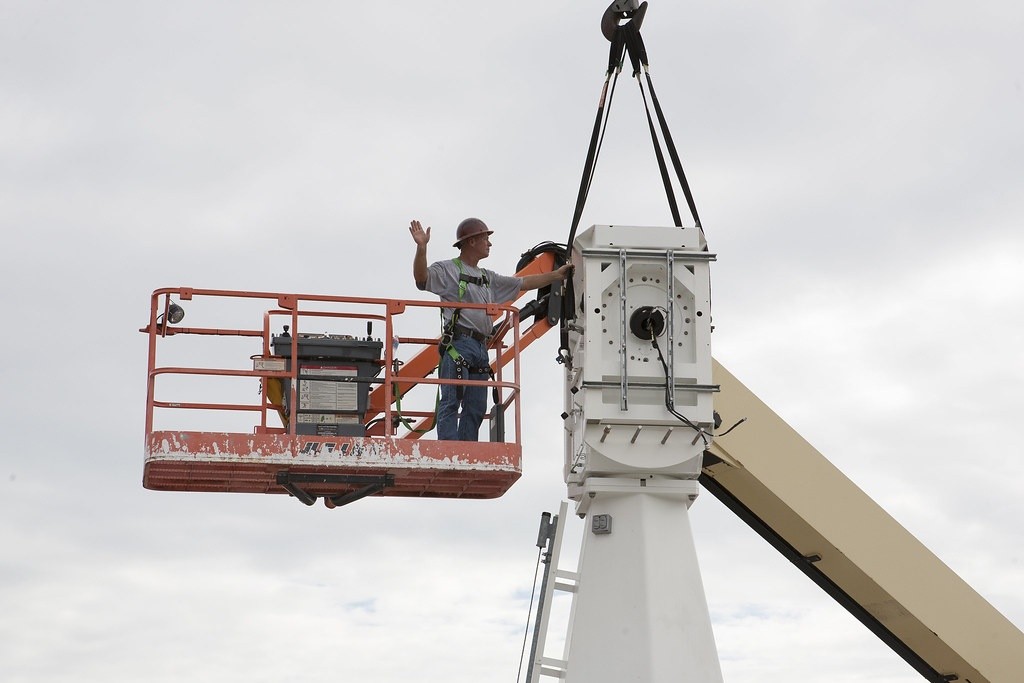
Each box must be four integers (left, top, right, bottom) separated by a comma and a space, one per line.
409, 218, 575, 442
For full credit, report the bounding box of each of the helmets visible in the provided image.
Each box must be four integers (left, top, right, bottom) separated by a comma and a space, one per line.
453, 218, 494, 247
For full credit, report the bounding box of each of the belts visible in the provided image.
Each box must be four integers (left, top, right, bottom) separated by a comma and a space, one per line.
455, 324, 486, 342
